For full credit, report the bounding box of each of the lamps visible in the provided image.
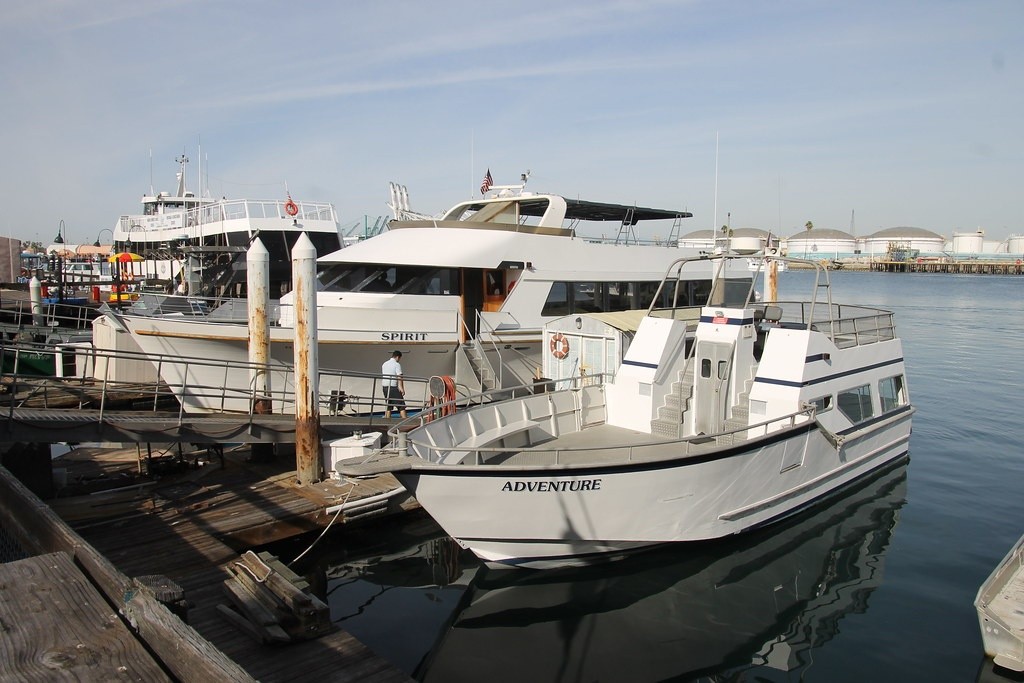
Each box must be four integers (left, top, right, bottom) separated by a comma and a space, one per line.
575, 317, 582, 330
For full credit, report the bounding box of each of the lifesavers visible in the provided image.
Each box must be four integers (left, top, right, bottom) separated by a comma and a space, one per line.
550, 334, 569, 358
284, 202, 298, 215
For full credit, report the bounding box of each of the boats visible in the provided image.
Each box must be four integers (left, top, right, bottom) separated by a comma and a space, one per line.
42, 262, 112, 290
110, 138, 341, 292
975, 534, 1024, 680
746, 258, 787, 272
98, 181, 758, 417
332, 233, 916, 575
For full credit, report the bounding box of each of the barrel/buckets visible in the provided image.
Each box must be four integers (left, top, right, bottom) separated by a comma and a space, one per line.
92, 286, 100, 300
41, 280, 47, 298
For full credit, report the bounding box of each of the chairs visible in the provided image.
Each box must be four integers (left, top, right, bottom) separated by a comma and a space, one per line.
756, 306, 783, 338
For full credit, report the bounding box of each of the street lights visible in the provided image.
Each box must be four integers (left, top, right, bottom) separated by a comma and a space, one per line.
55, 220, 69, 300
94, 230, 114, 295
128, 225, 148, 280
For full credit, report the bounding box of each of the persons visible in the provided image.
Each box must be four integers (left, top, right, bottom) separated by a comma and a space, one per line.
381, 350, 407, 418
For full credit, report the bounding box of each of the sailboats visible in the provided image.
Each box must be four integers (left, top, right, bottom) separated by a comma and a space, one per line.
831, 237, 843, 266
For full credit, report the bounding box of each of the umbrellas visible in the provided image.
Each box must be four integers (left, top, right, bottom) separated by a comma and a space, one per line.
107, 252, 145, 292
479, 170, 493, 195
